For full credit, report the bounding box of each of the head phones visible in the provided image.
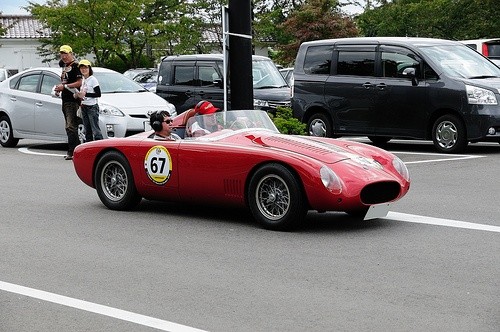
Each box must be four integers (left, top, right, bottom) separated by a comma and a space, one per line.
152, 114, 163, 132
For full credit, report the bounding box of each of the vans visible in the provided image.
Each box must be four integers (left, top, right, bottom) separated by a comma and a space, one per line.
291, 36, 500, 153
156, 55, 290, 115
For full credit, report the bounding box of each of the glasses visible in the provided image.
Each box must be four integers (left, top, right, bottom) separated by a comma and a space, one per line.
162, 119, 173, 124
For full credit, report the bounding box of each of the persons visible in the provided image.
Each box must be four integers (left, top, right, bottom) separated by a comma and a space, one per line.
53, 45, 83, 160
149, 110, 176, 141
73, 60, 104, 142
190, 100, 221, 136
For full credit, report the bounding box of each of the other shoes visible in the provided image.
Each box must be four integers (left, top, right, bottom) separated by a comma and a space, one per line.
64, 156, 72, 160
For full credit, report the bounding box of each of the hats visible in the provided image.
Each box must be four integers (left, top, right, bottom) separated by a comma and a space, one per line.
60, 45, 72, 54
78, 60, 92, 67
196, 101, 220, 115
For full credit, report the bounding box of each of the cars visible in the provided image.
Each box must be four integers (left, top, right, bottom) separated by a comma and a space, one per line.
123, 38, 500, 96
0, 67, 177, 148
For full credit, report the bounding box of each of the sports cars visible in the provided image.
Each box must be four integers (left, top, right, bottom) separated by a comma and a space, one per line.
73, 109, 412, 232
